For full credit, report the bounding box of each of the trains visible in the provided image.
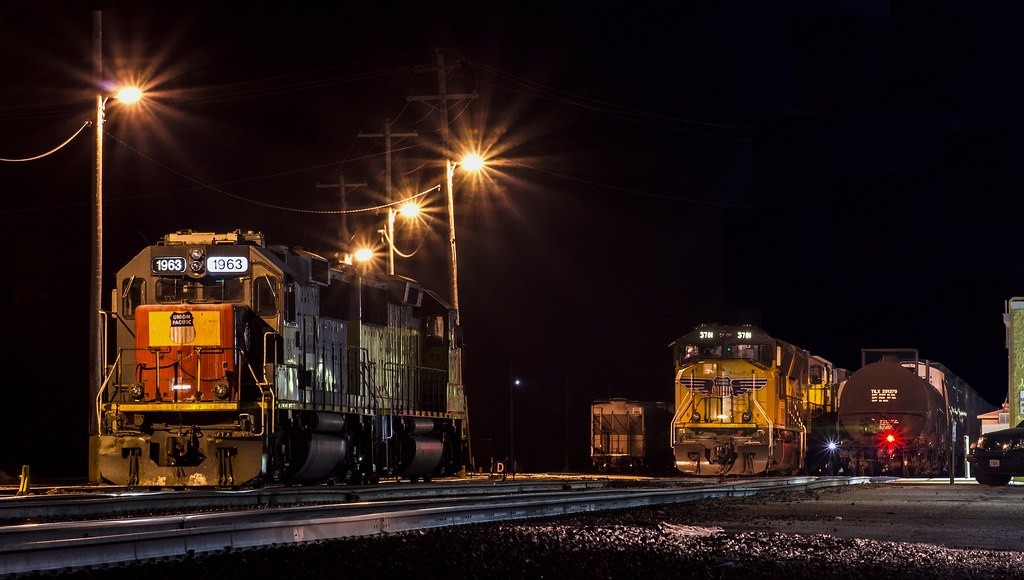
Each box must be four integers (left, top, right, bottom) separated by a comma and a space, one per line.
834, 347, 994, 477
84, 227, 470, 489
667, 323, 854, 478
590, 397, 674, 474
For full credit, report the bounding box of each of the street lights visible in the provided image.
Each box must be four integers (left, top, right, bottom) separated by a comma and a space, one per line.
388, 201, 421, 273
510, 381, 522, 474
445, 147, 485, 478
87, 85, 144, 487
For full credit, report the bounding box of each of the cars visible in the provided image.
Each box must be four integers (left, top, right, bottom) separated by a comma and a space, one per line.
966, 419, 1024, 486
